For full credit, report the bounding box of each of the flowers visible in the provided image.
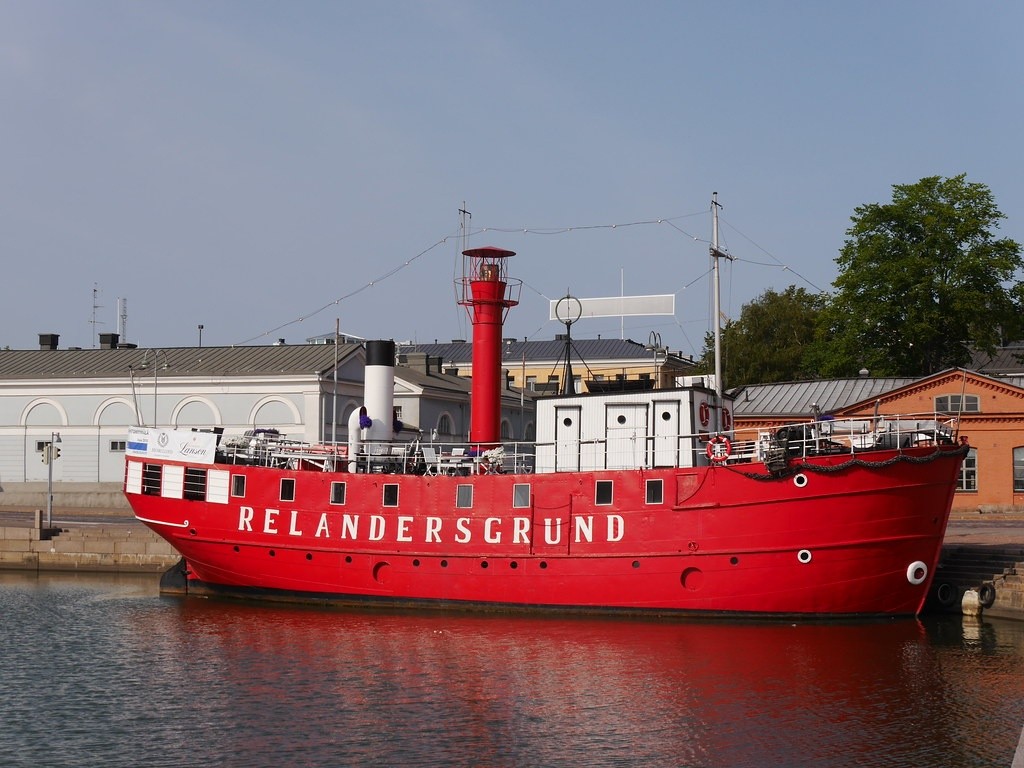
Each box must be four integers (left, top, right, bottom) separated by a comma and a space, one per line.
221, 435, 251, 448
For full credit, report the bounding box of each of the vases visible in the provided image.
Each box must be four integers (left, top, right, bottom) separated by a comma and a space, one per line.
225, 445, 241, 454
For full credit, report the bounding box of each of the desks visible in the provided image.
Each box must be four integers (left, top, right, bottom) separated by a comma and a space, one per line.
473, 456, 501, 475
269, 453, 345, 473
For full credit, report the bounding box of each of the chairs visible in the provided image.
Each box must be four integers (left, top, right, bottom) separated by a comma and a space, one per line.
423, 448, 534, 475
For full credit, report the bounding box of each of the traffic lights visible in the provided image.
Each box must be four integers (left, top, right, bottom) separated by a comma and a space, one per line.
41, 446, 48, 464
53, 447, 61, 459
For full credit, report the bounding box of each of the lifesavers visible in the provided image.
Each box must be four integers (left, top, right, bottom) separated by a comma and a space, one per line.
707, 436, 731, 461
936, 582, 958, 607
977, 583, 996, 606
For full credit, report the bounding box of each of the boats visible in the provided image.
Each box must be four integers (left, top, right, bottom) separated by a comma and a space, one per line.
118, 193, 972, 620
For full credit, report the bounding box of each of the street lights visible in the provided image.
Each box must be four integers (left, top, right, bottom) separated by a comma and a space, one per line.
644, 329, 666, 391
46, 432, 62, 529
139, 347, 171, 429
